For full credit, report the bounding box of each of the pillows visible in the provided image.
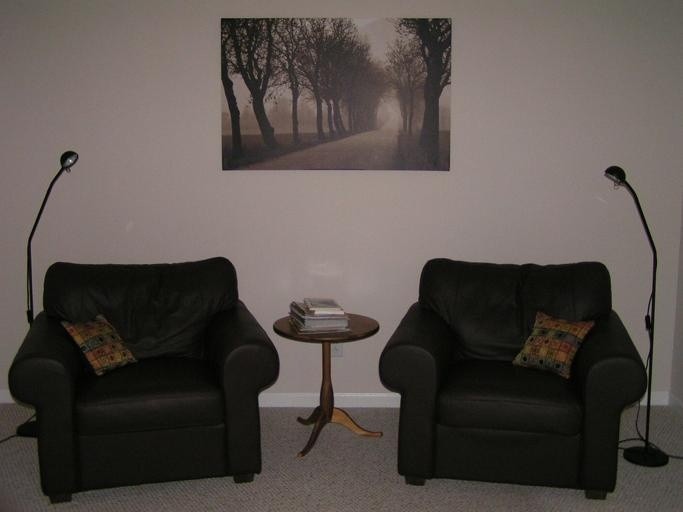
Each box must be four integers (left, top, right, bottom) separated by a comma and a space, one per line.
55, 313, 140, 377
509, 308, 595, 381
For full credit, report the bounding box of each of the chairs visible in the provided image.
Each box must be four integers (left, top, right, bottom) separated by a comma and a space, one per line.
378, 257, 648, 501
8, 256, 280, 505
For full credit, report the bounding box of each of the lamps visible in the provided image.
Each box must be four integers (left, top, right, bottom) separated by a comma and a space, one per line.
16, 150, 78, 437
604, 165, 670, 468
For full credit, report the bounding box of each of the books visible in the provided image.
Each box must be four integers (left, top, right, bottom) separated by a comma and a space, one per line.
288, 298, 351, 335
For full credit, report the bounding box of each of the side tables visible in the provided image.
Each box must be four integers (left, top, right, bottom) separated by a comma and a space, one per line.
273, 312, 384, 457
